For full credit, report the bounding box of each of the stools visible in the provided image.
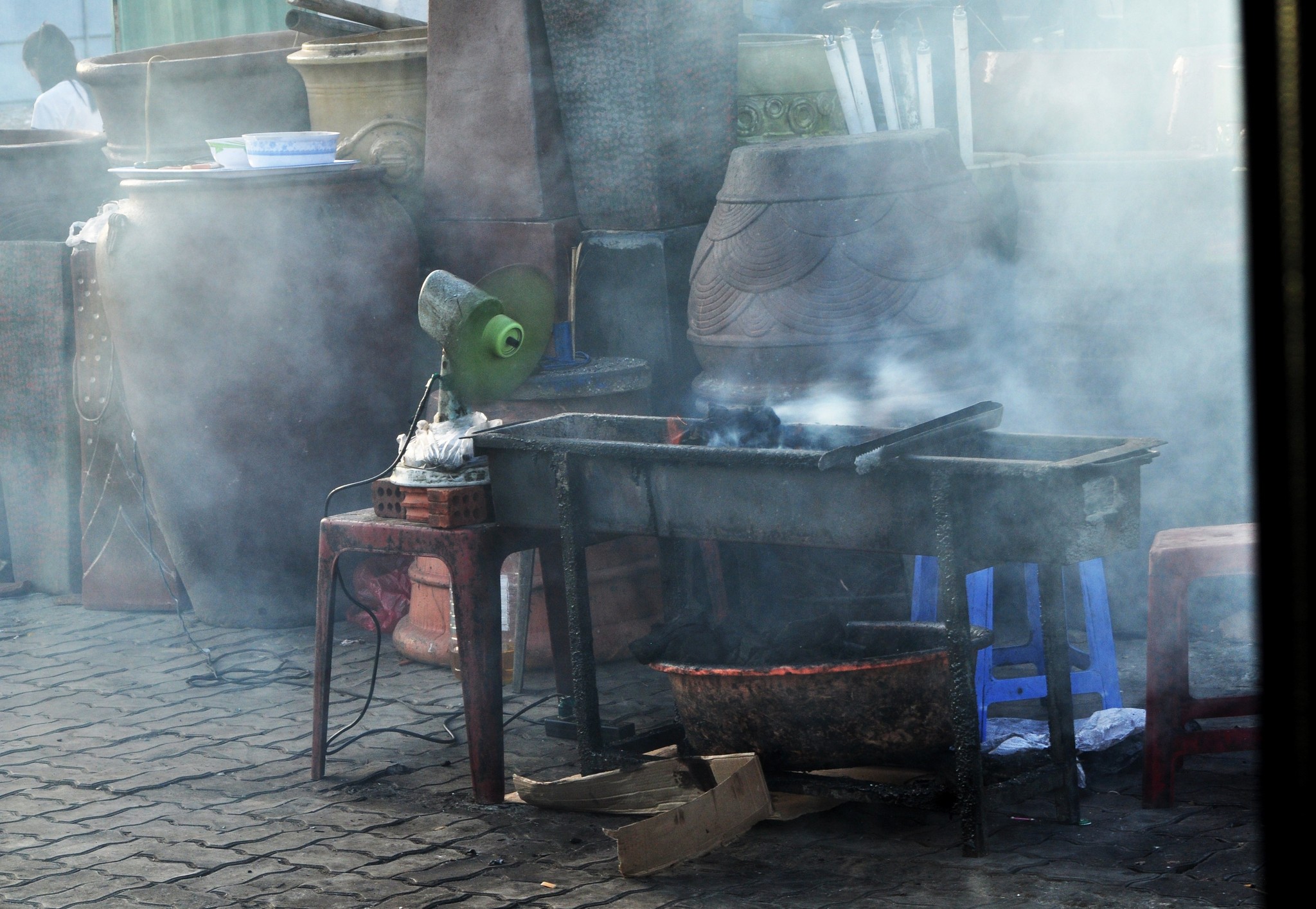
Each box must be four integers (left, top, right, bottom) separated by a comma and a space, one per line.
914, 555, 1122, 738
1144, 524, 1261, 806
312, 505, 568, 806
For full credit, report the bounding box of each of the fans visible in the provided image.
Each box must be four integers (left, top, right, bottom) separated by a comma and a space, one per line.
392, 262, 555, 485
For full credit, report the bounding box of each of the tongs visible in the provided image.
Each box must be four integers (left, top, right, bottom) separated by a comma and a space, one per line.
817, 400, 1003, 475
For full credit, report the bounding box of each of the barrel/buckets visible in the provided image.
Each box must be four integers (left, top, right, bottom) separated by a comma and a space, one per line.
94, 182, 427, 628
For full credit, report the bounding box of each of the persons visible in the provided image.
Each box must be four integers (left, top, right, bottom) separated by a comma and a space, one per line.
21, 24, 105, 133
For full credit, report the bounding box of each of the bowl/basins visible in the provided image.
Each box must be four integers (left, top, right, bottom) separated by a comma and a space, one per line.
205, 137, 252, 169
241, 131, 340, 168
76, 30, 310, 154
632, 619, 997, 766
287, 29, 425, 173
1, 128, 103, 240
